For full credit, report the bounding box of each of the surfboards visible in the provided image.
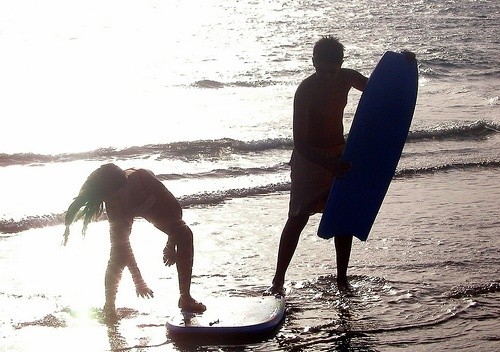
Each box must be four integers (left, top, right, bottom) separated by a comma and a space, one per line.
317, 50, 419, 241
166, 294, 287, 340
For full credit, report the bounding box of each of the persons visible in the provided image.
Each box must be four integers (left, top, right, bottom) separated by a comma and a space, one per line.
63, 163, 207, 312
261, 35, 371, 296
